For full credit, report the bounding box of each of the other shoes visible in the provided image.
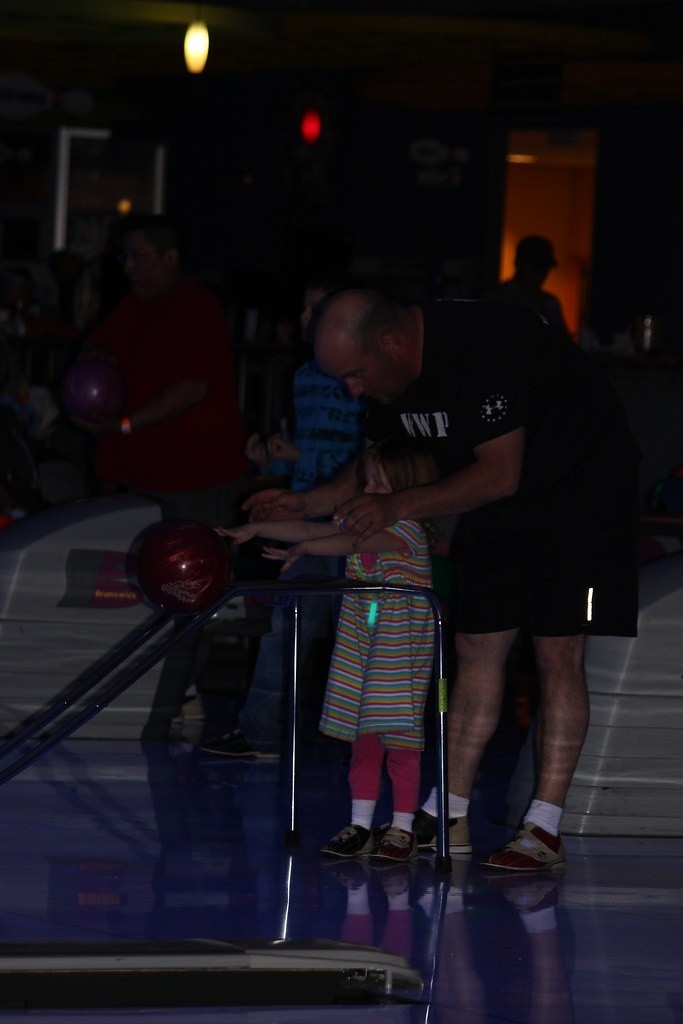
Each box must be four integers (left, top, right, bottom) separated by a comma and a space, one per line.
257, 733, 282, 757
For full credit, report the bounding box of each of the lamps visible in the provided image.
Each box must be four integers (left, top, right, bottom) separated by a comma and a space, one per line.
184, 1, 210, 75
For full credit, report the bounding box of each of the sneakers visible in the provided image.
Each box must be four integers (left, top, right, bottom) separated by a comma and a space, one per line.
478, 822, 568, 874
369, 828, 418, 861
199, 736, 262, 755
377, 808, 472, 853
320, 825, 375, 857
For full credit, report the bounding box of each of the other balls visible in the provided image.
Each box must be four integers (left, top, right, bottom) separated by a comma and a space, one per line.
128, 521, 231, 611
63, 350, 117, 412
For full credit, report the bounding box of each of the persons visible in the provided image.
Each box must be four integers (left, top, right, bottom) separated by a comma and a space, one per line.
76, 213, 642, 870
155, 842, 578, 1024
